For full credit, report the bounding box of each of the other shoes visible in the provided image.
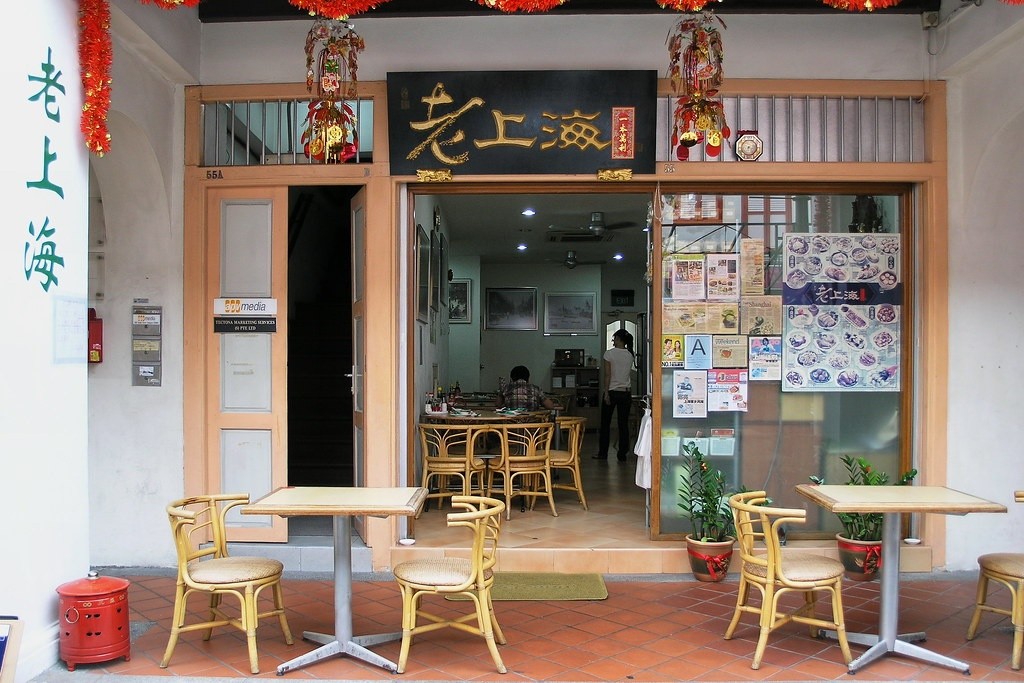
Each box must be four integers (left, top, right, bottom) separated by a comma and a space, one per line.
592, 454, 599, 458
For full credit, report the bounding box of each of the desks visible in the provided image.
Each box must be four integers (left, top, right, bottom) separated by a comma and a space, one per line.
240, 487, 431, 674
796, 484, 1008, 676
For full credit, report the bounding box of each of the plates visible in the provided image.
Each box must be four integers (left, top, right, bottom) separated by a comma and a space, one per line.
429, 411, 448, 414
786, 235, 899, 290
450, 412, 470, 417
785, 307, 898, 387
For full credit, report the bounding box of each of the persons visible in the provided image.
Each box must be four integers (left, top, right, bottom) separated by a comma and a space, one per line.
590, 329, 635, 462
677, 376, 692, 390
494, 365, 560, 482
663, 339, 682, 355
760, 338, 776, 354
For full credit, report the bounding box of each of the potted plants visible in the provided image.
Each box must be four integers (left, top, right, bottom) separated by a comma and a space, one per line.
681, 441, 772, 582
809, 454, 919, 582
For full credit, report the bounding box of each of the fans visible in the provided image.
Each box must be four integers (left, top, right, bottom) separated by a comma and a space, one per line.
546, 211, 639, 237
539, 251, 606, 269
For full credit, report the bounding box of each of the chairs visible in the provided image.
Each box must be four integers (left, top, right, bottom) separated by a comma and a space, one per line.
724, 490, 855, 670
161, 496, 295, 674
966, 491, 1024, 670
395, 497, 505, 674
415, 394, 588, 517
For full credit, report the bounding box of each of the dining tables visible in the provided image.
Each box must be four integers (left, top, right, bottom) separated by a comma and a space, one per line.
418, 408, 551, 513
456, 399, 562, 479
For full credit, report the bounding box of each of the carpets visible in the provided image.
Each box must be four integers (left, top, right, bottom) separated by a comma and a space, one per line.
445, 572, 608, 600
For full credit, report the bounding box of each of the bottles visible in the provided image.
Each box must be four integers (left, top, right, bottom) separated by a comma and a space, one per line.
424, 381, 461, 413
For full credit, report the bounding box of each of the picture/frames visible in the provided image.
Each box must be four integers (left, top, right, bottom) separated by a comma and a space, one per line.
484, 287, 538, 331
415, 224, 448, 324
544, 291, 598, 334
447, 278, 472, 323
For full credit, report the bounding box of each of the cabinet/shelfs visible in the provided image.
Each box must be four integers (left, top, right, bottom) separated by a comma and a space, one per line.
550, 366, 602, 434
